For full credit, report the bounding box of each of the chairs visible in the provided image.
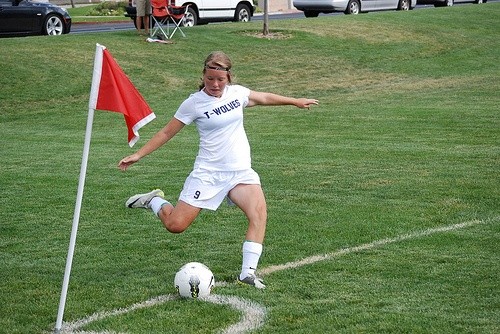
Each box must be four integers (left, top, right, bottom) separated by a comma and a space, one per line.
149, 0, 193, 40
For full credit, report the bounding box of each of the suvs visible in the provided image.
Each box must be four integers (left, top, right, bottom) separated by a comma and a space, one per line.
125, 0, 256, 29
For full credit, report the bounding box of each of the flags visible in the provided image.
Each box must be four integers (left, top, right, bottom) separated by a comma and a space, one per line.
90, 43, 156, 148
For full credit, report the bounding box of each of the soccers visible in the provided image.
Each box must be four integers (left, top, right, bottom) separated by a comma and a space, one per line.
174, 261, 215, 299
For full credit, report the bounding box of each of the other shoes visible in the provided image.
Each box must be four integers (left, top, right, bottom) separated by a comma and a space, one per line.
138, 32, 153, 35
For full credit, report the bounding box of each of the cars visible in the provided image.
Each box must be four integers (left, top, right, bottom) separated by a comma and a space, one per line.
416, 0, 487, 8
0, 0, 72, 38
293, 0, 417, 17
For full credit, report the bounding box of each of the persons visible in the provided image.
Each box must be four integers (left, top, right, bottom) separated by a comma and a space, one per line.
134, 0, 153, 32
117, 50, 319, 290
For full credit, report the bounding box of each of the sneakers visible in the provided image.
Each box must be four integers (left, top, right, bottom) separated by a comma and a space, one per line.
238, 272, 267, 289
125, 189, 164, 209
